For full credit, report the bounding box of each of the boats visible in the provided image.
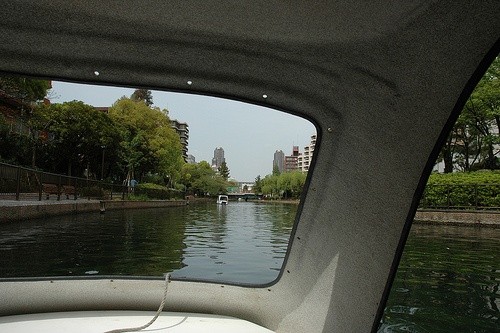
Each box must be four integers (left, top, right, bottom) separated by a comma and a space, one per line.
216, 194, 229, 205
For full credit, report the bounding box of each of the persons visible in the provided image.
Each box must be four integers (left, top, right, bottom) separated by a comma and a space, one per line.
131, 180, 139, 192
